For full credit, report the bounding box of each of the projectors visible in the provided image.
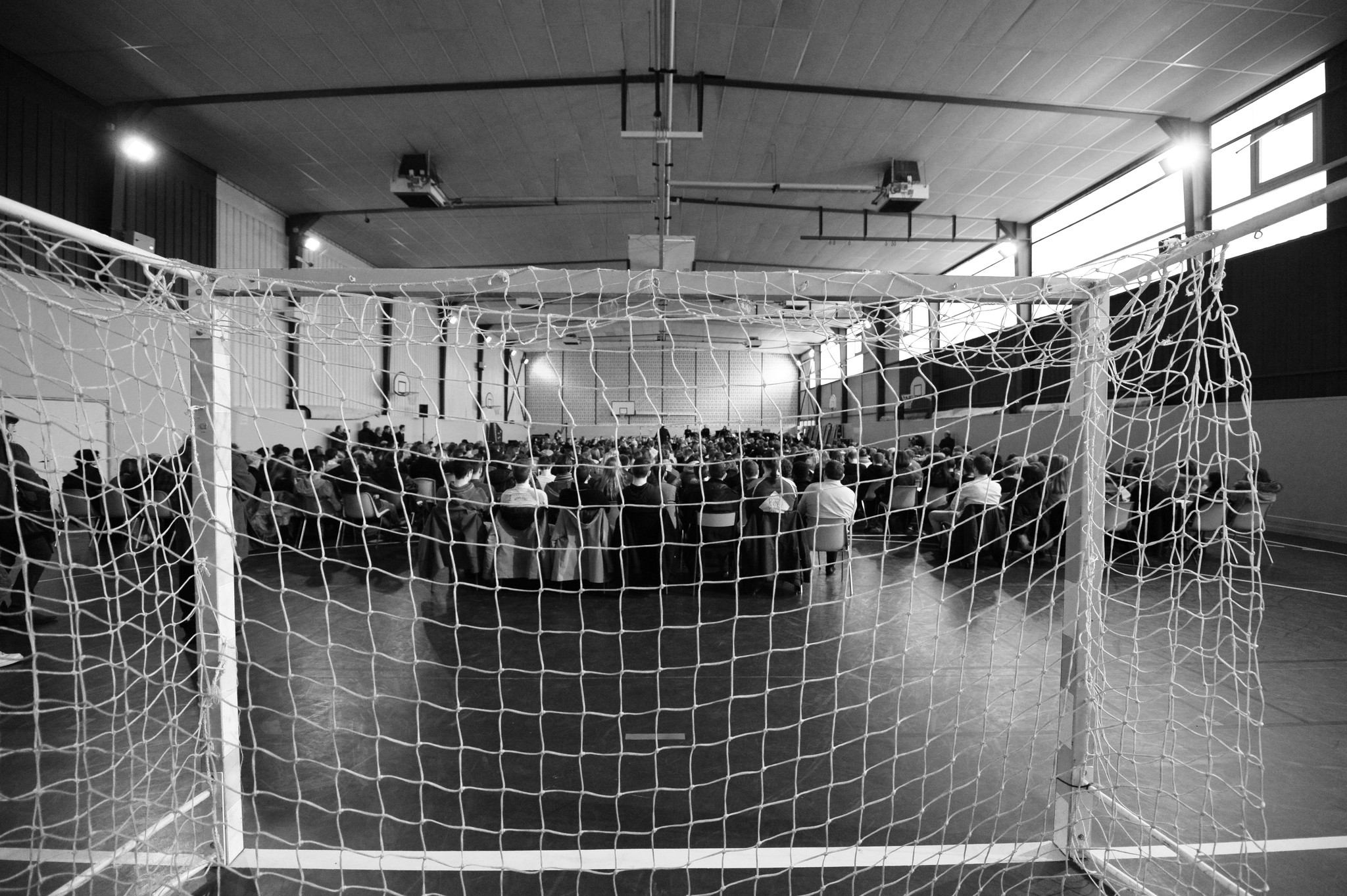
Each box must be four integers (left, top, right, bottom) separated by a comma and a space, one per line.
870, 182, 930, 213
389, 179, 446, 209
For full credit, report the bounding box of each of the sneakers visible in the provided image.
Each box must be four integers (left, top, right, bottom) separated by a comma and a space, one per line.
0, 651, 24, 668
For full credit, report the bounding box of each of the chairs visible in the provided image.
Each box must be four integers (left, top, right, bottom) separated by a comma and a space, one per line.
1227, 501, 1274, 564
412, 478, 438, 504
800, 516, 853, 598
97, 489, 139, 549
1185, 502, 1238, 565
342, 492, 393, 547
55, 490, 102, 548
300, 494, 356, 548
1105, 500, 1149, 568
919, 484, 951, 547
691, 511, 738, 595
135, 490, 177, 550
877, 485, 922, 537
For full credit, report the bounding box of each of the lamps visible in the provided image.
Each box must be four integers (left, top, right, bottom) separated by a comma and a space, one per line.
447, 315, 457, 324
523, 358, 528, 365
509, 349, 517, 356
484, 335, 491, 343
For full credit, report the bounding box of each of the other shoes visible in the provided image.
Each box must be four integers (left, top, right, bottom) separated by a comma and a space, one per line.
1026, 558, 1035, 566
139, 534, 151, 541
1190, 541, 1206, 551
7, 605, 57, 624
825, 564, 836, 576
235, 625, 241, 632
992, 558, 1008, 565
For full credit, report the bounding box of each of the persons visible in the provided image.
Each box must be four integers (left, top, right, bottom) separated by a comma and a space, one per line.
0, 410, 1274, 666
